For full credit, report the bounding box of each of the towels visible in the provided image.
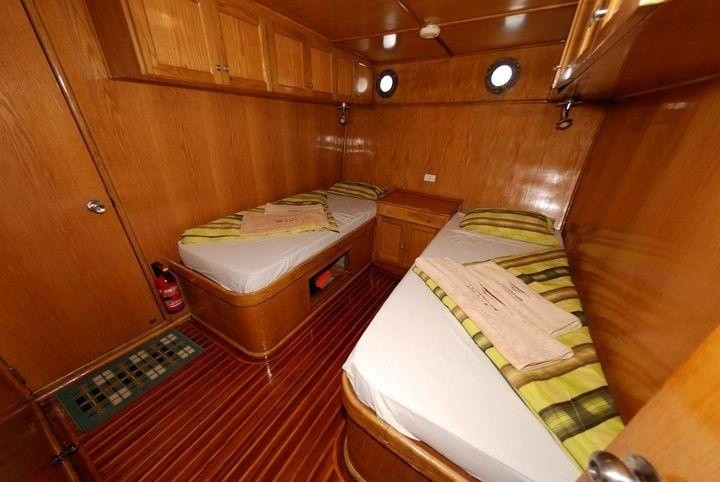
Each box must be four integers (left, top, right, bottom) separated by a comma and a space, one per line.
240, 209, 328, 233
415, 256, 574, 370
264, 202, 322, 212
468, 261, 583, 336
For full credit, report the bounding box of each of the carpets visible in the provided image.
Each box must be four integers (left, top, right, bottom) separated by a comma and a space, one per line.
54, 327, 203, 432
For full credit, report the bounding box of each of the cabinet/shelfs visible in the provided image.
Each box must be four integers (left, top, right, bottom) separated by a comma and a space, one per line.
86, 0, 372, 105
547, 0, 720, 102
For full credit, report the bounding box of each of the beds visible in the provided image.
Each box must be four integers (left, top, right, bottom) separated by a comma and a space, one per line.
168, 185, 393, 362
333, 206, 625, 481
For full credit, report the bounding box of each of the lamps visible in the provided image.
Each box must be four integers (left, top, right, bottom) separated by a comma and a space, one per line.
337, 102, 348, 123
420, 24, 440, 39
555, 103, 573, 130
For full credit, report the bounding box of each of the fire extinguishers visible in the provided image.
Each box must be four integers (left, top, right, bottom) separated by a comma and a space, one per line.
151, 261, 185, 314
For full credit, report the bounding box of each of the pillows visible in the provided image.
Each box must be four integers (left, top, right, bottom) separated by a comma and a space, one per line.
326, 179, 389, 200
461, 208, 560, 246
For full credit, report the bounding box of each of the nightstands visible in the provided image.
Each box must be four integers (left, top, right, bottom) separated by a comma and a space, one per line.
372, 189, 463, 269
